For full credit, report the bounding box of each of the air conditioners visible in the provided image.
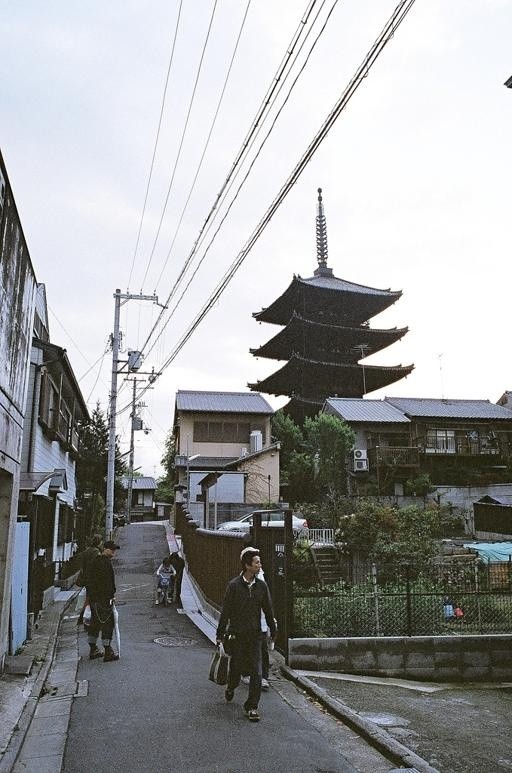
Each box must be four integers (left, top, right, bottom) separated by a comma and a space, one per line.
353, 449, 370, 473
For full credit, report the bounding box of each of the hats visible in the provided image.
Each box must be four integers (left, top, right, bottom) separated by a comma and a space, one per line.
103, 540, 120, 551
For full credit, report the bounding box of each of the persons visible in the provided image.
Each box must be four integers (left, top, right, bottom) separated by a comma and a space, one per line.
216, 550, 278, 722
86, 540, 120, 663
240, 546, 278, 689
169, 552, 185, 608
76, 533, 102, 625
155, 557, 177, 605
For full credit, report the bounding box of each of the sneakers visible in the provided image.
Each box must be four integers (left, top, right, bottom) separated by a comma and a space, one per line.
225, 684, 240, 701
261, 679, 268, 689
156, 597, 173, 604
241, 704, 261, 721
241, 674, 250, 685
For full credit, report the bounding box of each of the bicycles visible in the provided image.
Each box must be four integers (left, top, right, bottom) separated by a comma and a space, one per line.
155, 571, 175, 607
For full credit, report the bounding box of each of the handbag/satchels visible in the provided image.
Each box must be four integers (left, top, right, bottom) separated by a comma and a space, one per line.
208, 642, 230, 685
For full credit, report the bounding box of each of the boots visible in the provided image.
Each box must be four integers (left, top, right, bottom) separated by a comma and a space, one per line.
105, 647, 119, 661
89, 645, 105, 660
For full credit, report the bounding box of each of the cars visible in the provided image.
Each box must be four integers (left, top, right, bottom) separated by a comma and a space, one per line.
211, 507, 311, 542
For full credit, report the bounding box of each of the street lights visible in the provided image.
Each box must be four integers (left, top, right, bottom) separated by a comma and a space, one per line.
186, 431, 201, 508
